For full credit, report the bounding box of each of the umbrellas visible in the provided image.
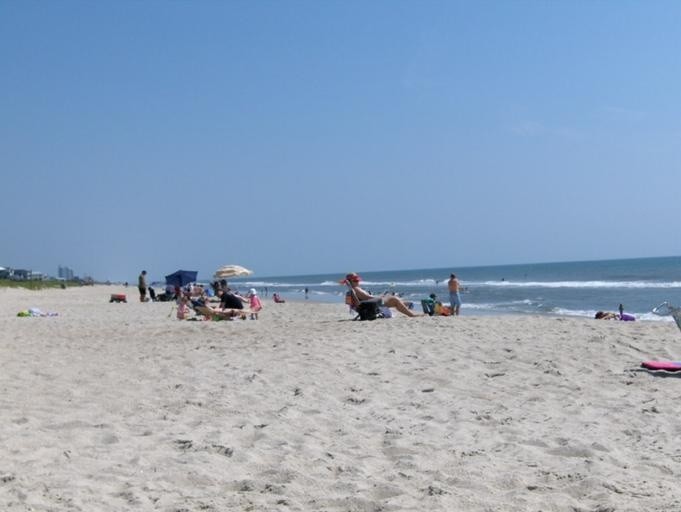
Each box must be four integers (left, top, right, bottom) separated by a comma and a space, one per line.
211, 263, 255, 280
165, 269, 199, 287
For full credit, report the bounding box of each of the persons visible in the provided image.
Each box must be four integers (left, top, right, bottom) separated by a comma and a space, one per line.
273, 292, 286, 303
597, 309, 618, 318
164, 279, 263, 321
432, 293, 442, 306
448, 272, 463, 315
341, 271, 430, 317
137, 270, 149, 302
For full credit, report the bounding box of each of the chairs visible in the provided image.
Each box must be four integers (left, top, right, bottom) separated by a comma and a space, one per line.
345, 279, 385, 321
